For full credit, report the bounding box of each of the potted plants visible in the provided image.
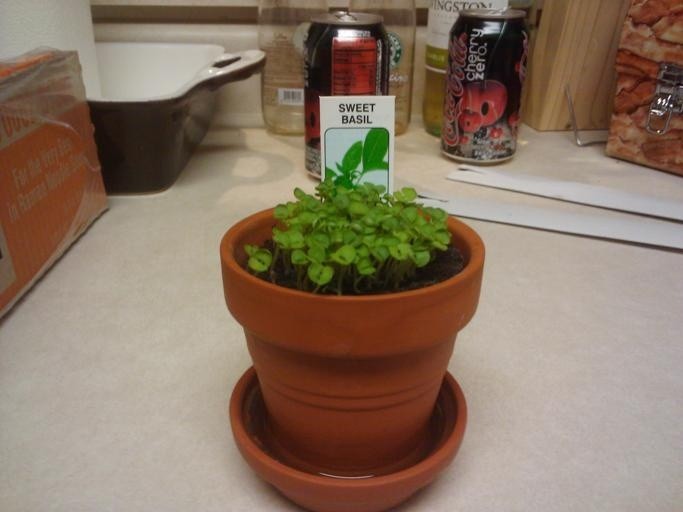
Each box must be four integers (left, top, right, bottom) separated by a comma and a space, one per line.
221, 170, 485, 510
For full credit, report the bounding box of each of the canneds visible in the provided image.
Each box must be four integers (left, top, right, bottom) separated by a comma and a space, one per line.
303, 6, 391, 182
440, 8, 530, 164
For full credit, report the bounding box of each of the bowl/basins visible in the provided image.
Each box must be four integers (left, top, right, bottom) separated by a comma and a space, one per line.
83, 40, 269, 197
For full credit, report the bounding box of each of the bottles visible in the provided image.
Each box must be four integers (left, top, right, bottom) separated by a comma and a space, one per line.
256, 0, 329, 138
349, 0, 418, 138
421, 0, 511, 138
